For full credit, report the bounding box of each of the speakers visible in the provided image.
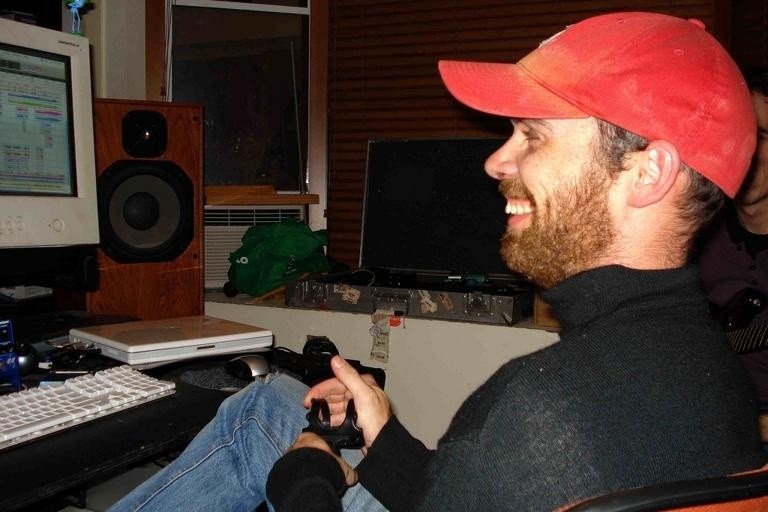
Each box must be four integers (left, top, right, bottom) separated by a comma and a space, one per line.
85, 95, 206, 320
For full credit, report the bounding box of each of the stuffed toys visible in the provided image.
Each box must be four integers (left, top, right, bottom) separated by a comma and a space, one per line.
221, 215, 330, 299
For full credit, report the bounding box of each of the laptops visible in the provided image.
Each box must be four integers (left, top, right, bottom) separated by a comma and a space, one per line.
69, 315, 273, 365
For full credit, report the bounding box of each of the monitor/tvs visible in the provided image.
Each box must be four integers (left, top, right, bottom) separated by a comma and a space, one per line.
359, 136, 524, 283
0, 17, 104, 251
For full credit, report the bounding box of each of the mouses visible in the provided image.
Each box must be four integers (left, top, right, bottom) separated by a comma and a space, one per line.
224, 353, 271, 379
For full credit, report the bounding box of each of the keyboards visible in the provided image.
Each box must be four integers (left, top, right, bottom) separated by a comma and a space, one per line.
0, 364, 177, 449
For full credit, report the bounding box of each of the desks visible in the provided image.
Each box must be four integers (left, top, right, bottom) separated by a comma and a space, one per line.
0, 349, 304, 512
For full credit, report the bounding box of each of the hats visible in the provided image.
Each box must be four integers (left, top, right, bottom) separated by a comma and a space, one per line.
436, 11, 759, 199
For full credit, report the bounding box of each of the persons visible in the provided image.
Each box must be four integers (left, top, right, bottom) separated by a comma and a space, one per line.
104, 11, 766, 511
688, 52, 767, 445
